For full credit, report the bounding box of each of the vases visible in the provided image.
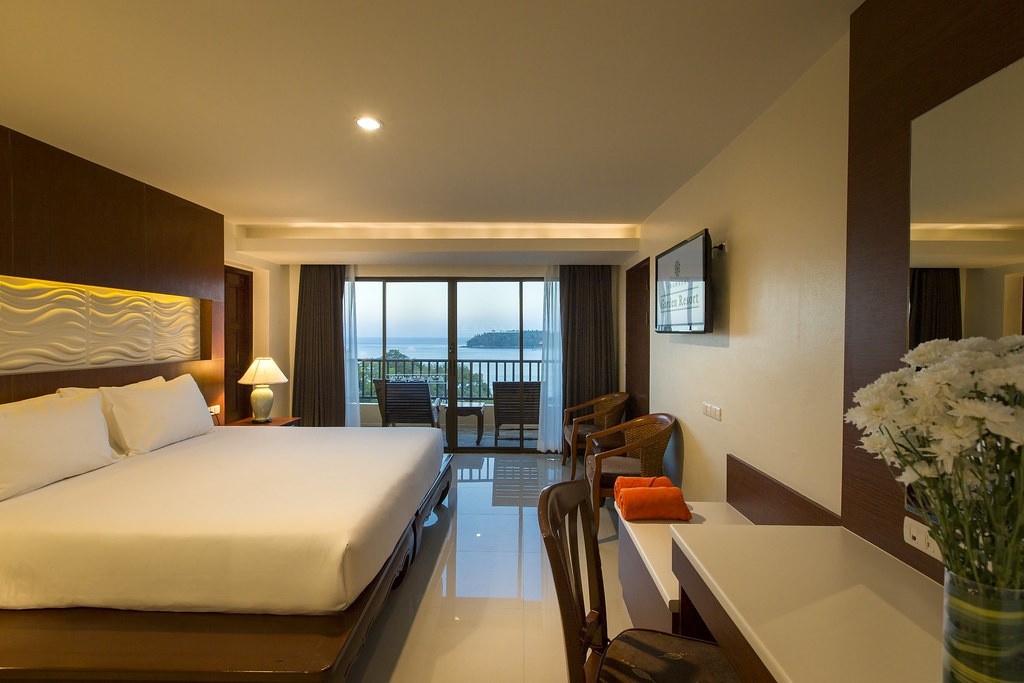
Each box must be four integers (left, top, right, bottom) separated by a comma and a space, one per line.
942, 567, 1024, 683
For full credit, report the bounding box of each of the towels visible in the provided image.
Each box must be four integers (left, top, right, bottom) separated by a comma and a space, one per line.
613, 474, 693, 520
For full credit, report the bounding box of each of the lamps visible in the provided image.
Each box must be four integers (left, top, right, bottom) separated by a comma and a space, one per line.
237, 357, 289, 424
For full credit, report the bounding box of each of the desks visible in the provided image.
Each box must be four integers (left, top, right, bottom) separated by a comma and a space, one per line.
587, 431, 627, 457
444, 401, 485, 445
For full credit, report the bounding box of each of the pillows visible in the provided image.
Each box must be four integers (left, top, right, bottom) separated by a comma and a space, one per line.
56, 375, 165, 453
0, 390, 120, 500
98, 373, 217, 457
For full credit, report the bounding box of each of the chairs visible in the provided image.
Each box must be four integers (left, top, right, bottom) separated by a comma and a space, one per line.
584, 413, 675, 507
493, 382, 542, 446
563, 392, 629, 480
537, 480, 740, 683
373, 378, 440, 428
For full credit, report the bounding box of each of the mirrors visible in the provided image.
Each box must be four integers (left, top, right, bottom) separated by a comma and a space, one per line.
909, 56, 1024, 352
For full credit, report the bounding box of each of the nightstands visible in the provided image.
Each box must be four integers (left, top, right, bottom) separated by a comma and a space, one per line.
0, 425, 443, 613
226, 417, 302, 426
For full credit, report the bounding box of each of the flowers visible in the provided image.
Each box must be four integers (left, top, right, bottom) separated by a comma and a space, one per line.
844, 335, 1024, 511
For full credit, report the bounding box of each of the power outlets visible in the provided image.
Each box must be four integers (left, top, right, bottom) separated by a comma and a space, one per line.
207, 405, 220, 416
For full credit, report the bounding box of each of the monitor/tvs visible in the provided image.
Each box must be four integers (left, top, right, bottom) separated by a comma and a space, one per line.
654, 227, 712, 335
